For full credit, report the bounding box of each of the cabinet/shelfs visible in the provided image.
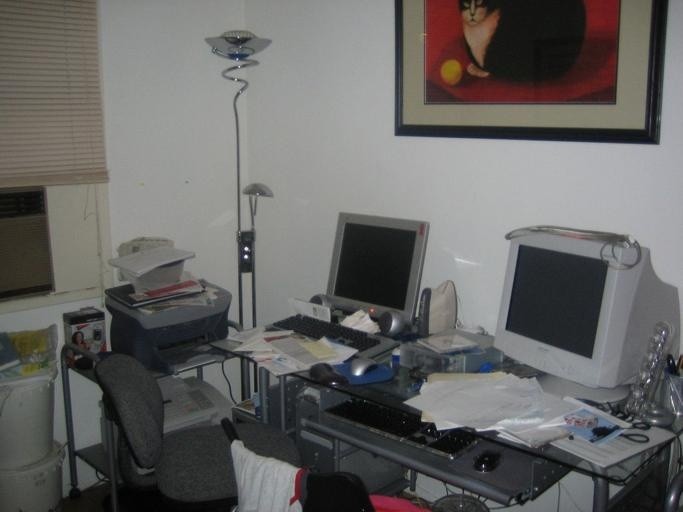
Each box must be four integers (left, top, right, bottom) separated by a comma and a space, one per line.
62, 320, 248, 511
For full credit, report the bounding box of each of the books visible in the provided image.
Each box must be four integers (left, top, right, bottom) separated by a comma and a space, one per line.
261, 334, 342, 371
497, 397, 677, 469
416, 333, 477, 354
104, 277, 220, 315
0, 331, 21, 372
108, 244, 196, 277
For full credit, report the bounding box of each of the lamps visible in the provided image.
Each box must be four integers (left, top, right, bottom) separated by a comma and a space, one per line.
205, 32, 271, 400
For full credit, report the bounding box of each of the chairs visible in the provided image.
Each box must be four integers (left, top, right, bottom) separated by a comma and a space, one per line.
220, 417, 430, 512
94, 354, 238, 511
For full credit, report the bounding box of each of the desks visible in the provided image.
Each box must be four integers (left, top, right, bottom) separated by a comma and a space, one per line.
208, 315, 683, 512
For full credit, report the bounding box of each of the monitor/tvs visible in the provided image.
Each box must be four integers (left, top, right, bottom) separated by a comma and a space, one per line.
492, 231, 681, 405
327, 212, 431, 324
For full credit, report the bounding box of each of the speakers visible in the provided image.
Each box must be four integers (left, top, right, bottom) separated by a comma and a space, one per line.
378, 311, 404, 338
309, 294, 332, 310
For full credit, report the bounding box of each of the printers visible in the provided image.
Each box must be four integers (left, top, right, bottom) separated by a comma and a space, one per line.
105, 282, 232, 374
101, 376, 230, 459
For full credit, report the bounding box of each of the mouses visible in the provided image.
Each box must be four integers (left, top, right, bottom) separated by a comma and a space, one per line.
349, 357, 377, 377
472, 450, 500, 472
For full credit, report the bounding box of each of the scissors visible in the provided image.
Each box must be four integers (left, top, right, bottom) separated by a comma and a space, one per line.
618, 422, 650, 442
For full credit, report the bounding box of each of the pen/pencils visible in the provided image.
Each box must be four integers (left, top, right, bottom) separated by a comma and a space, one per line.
665, 354, 683, 415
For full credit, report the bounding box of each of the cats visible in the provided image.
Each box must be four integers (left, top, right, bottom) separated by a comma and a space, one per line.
459, 0, 587, 83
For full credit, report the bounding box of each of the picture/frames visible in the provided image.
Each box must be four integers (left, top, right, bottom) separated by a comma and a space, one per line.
395, 0, 669, 147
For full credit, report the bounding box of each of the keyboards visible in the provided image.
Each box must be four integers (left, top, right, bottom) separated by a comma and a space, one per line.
324, 396, 483, 461
265, 313, 400, 359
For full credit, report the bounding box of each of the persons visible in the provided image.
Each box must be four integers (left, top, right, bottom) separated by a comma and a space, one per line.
71, 330, 90, 356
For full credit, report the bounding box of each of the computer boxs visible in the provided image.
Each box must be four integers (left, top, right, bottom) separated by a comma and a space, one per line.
294, 381, 405, 496
